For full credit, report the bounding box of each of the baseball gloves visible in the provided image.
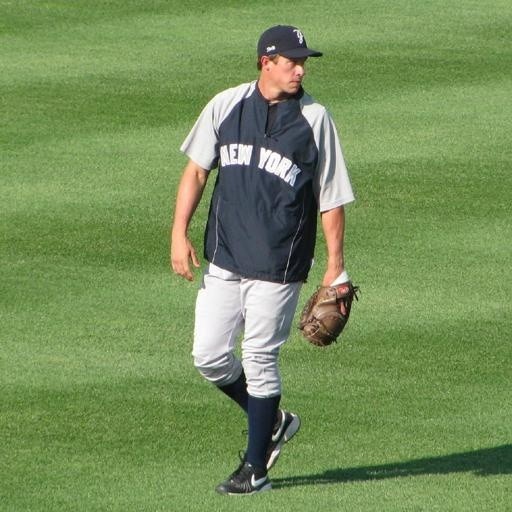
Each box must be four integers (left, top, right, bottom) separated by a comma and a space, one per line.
295, 270, 359, 346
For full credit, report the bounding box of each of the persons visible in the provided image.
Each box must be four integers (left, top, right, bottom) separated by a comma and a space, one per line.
170, 25, 356, 498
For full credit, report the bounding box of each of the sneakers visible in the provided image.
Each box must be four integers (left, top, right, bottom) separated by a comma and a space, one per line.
266, 408, 301, 471
215, 460, 273, 497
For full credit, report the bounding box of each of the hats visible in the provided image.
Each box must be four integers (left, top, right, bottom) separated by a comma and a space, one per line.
257, 25, 323, 60
